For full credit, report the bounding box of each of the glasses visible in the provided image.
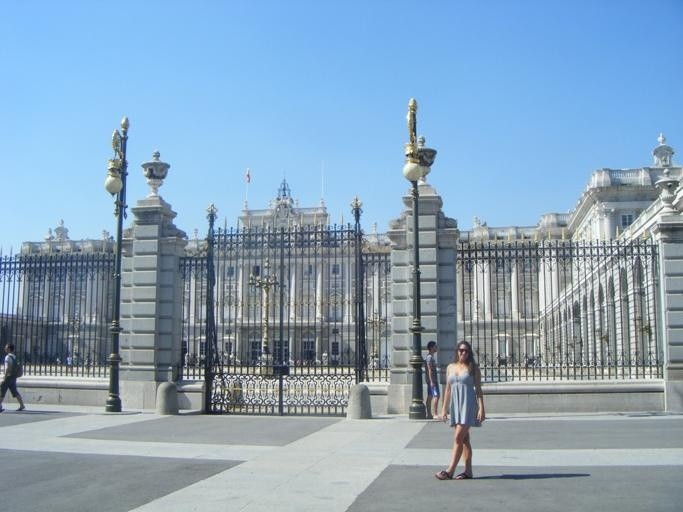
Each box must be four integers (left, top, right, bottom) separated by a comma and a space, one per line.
458, 348, 470, 353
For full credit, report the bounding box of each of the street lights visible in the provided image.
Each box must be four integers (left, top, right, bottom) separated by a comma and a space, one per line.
71, 319, 81, 366
247, 257, 279, 376
404, 95, 436, 419
368, 309, 387, 371
103, 116, 127, 413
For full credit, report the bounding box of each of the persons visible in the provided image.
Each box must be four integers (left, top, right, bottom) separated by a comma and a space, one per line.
0, 342, 24, 412
423, 340, 440, 419
433, 341, 485, 480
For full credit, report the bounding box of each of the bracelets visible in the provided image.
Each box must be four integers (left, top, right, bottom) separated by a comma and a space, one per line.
476, 395, 483, 397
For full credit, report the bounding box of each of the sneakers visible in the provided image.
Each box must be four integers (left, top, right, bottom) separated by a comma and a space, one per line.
426, 416, 433, 419
433, 416, 442, 420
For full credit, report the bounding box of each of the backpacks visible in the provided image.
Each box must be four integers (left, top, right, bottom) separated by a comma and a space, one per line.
7, 354, 23, 378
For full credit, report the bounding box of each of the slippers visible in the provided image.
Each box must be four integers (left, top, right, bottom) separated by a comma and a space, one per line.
15, 406, 25, 412
455, 473, 473, 481
434, 471, 453, 481
0, 408, 5, 413
